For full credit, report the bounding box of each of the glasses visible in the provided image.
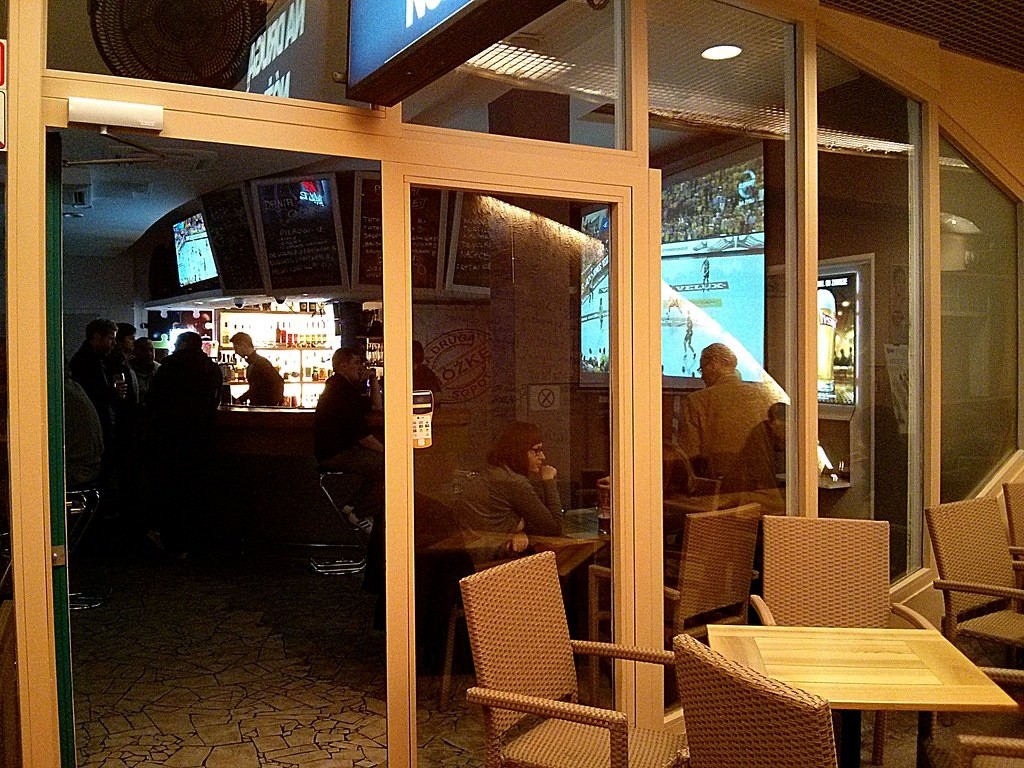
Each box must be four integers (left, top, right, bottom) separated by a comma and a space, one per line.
531, 447, 544, 456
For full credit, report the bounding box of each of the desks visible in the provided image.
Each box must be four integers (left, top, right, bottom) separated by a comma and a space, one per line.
702, 624, 1021, 768
511, 505, 676, 559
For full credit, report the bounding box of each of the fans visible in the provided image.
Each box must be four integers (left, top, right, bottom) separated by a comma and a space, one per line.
89, 0, 267, 94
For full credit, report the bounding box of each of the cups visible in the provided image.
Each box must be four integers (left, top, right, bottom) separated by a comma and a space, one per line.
816, 289, 838, 391
114, 372, 125, 391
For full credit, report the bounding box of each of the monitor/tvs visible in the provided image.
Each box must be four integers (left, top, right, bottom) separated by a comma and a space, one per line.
172, 212, 221, 291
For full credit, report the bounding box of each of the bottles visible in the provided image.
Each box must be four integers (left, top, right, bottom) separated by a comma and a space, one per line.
221, 297, 384, 409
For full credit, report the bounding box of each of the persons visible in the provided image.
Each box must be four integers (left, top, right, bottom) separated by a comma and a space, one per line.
105, 320, 141, 421
734, 400, 789, 495
850, 405, 907, 527
130, 335, 163, 398
671, 344, 781, 495
136, 332, 224, 480
230, 332, 286, 407
312, 346, 385, 536
445, 420, 564, 558
61, 352, 105, 496
412, 339, 444, 408
65, 317, 129, 524
592, 417, 697, 498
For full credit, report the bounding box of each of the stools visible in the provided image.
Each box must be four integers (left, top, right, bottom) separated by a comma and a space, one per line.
64, 490, 108, 611
309, 462, 373, 575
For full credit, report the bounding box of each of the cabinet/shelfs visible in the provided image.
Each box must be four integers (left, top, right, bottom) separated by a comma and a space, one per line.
361, 336, 386, 395
212, 308, 342, 408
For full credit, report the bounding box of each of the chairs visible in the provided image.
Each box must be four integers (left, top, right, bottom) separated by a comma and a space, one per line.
749, 513, 943, 765
585, 502, 760, 715
673, 635, 841, 768
922, 499, 1024, 728
457, 551, 689, 768
